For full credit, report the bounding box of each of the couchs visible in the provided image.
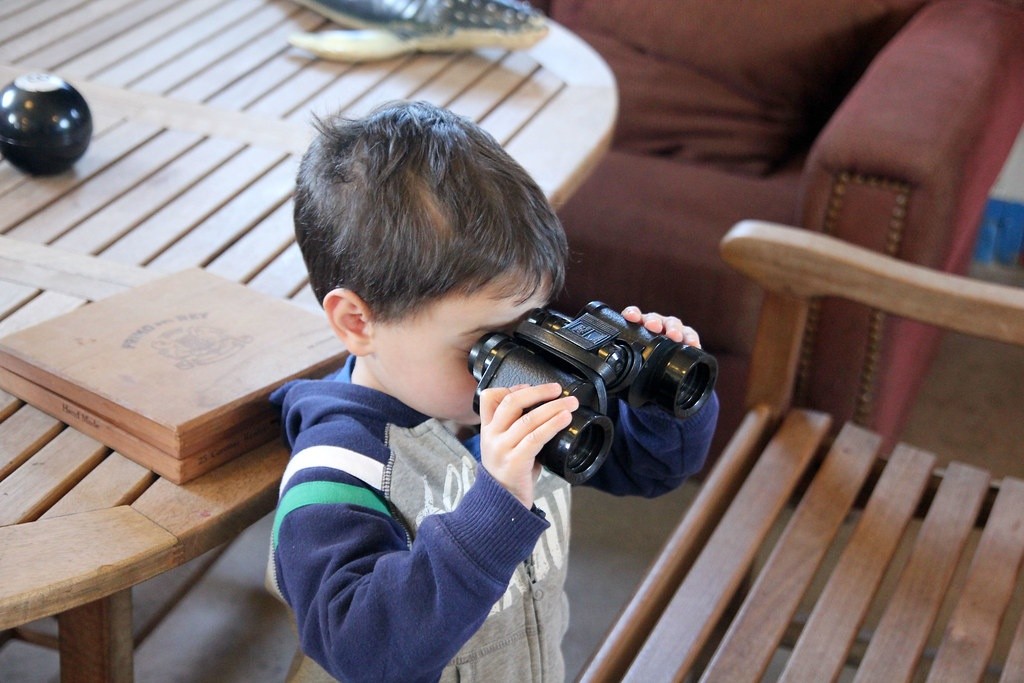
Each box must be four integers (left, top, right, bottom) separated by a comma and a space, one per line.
528, 1, 1024, 473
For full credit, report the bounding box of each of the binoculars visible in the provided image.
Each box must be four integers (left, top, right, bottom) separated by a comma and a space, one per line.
466, 300, 719, 486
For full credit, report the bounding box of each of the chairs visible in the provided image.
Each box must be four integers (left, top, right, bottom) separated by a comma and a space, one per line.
571, 217, 1024, 682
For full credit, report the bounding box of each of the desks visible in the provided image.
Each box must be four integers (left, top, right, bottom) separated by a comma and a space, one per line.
0, 1, 624, 633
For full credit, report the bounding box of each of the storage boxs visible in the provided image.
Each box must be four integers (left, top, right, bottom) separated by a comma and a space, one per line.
0, 266, 346, 487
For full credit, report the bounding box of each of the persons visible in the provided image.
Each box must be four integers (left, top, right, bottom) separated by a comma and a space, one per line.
266, 98, 719, 683
